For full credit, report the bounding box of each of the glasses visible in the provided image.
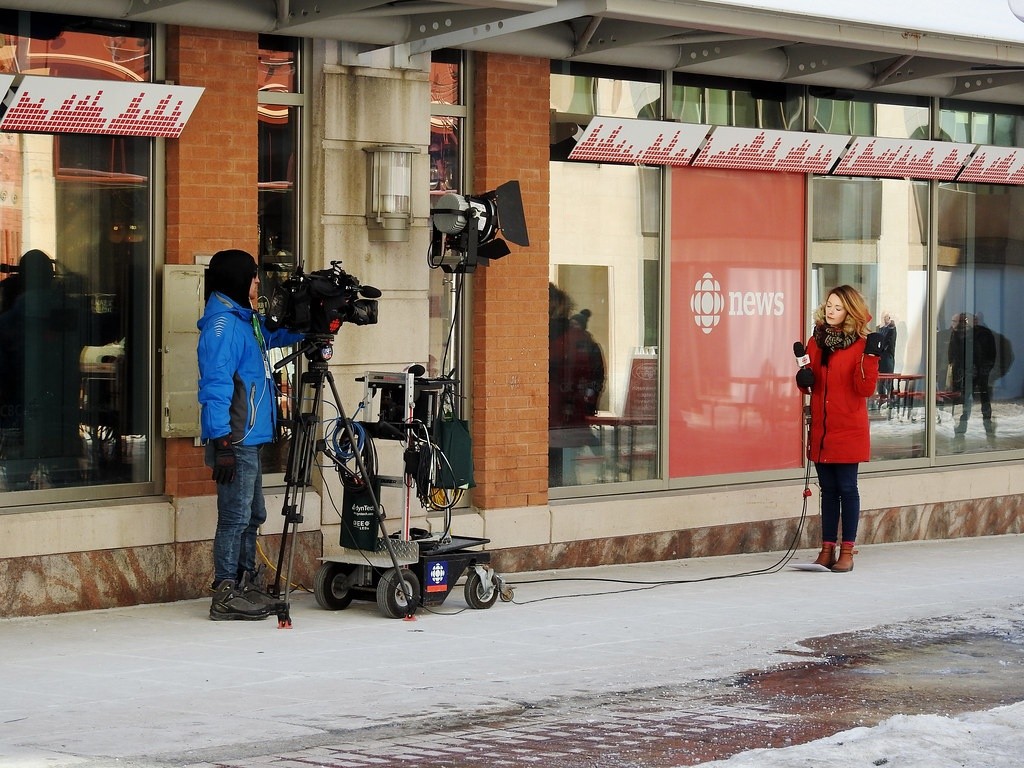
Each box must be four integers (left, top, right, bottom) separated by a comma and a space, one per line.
253, 269, 258, 280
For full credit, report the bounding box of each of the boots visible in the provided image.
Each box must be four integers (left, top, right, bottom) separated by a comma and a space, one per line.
814, 543, 837, 568
832, 544, 854, 571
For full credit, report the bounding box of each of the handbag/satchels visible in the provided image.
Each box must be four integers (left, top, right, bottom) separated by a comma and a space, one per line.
433, 388, 478, 490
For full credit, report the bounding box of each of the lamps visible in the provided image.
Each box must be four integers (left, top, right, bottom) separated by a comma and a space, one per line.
359, 139, 423, 247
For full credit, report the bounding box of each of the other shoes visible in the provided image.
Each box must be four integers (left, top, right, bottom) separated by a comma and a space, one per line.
955, 423, 967, 434
983, 420, 992, 433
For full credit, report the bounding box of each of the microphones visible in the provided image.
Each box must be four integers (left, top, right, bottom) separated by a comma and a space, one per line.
794, 342, 812, 393
351, 286, 382, 298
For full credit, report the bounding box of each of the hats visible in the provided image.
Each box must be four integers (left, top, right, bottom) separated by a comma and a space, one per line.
571, 309, 590, 328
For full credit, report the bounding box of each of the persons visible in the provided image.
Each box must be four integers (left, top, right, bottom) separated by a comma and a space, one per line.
796, 285, 886, 572
548, 286, 606, 486
0, 249, 116, 491
196, 249, 303, 620
936, 312, 997, 432
874, 312, 895, 408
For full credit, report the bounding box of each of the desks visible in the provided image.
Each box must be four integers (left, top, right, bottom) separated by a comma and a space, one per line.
866, 371, 924, 421
589, 414, 659, 482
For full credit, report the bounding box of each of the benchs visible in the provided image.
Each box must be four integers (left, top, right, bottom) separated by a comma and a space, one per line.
870, 387, 962, 419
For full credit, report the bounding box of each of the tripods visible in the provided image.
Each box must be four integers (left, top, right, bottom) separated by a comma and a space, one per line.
270, 333, 416, 629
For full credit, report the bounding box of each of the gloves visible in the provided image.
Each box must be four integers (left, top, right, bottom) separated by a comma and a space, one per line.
211, 433, 237, 487
865, 332, 884, 357
796, 367, 815, 389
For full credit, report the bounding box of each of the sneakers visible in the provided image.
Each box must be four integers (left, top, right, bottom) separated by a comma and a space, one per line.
208, 578, 272, 621
236, 571, 283, 615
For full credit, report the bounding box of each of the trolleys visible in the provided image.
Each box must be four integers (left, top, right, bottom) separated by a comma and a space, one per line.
314, 415, 514, 619
